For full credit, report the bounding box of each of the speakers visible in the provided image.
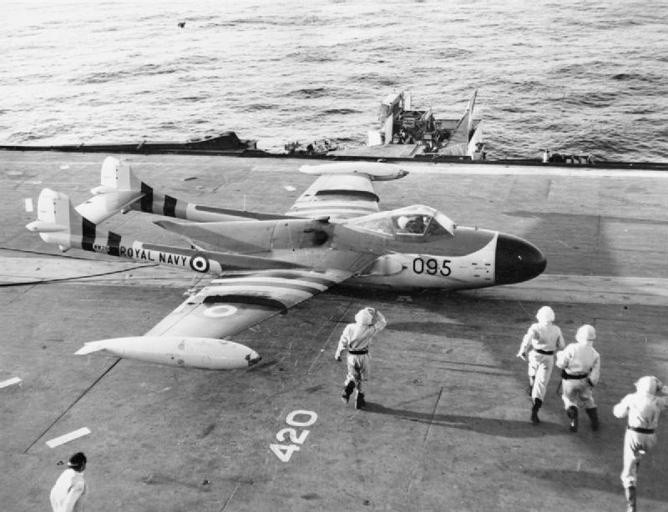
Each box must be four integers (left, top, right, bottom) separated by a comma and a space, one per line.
531, 398, 542, 423
585, 408, 600, 430
342, 381, 355, 403
625, 485, 636, 512
567, 406, 577, 432
357, 392, 364, 409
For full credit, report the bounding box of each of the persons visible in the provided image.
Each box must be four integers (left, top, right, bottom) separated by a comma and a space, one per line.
611, 374, 666, 511
554, 323, 601, 433
49, 451, 87, 511
515, 305, 566, 423
334, 307, 388, 409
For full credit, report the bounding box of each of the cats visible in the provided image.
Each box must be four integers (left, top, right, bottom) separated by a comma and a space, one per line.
22, 154, 550, 374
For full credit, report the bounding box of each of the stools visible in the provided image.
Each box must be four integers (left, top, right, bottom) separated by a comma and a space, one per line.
348, 351, 366, 354
566, 374, 587, 379
627, 427, 655, 433
536, 350, 554, 355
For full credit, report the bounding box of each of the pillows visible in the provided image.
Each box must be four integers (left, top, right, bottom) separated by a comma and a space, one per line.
535, 306, 555, 322
354, 309, 373, 325
633, 376, 658, 394
574, 325, 596, 341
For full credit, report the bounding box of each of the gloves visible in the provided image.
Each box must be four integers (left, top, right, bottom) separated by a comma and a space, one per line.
364, 84, 492, 162
